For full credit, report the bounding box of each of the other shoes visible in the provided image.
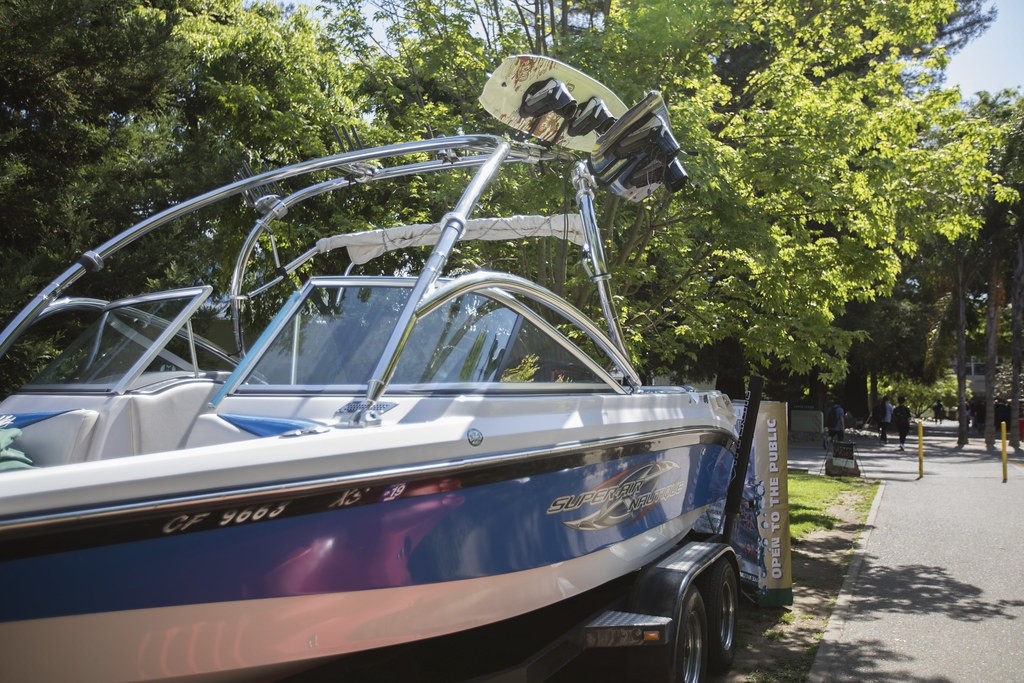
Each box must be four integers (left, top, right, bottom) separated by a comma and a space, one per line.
900, 444, 904, 451
823, 439, 827, 449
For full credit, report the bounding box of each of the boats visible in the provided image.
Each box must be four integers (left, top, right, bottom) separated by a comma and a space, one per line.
0, 55, 765, 683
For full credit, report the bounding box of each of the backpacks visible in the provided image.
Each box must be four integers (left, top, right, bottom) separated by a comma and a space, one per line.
827, 406, 840, 427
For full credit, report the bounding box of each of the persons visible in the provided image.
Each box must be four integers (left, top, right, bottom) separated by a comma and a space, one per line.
933, 400, 944, 424
958, 398, 970, 431
878, 395, 894, 444
823, 399, 845, 450
892, 396, 911, 452
